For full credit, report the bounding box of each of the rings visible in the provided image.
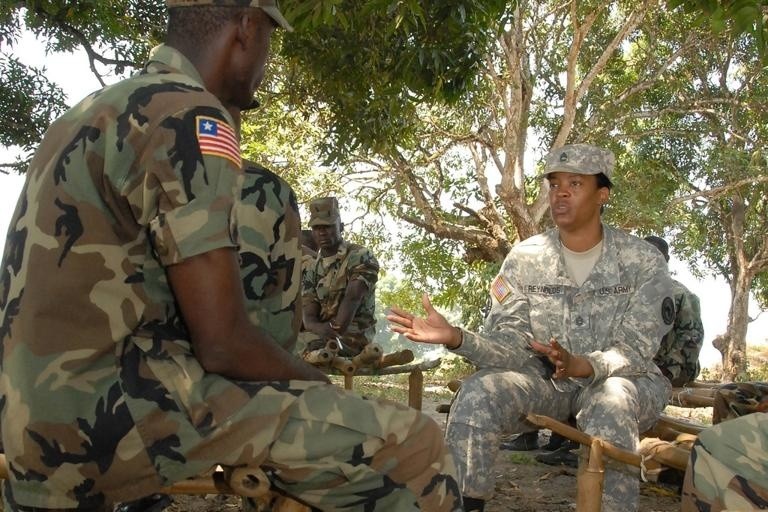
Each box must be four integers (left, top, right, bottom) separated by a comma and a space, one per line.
558, 360, 569, 372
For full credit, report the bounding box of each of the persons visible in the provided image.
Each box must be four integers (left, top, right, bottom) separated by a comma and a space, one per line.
298, 196, 380, 361
676, 410, 766, 512
535, 233, 705, 468
500, 414, 574, 453
293, 228, 321, 260
231, 152, 304, 395
710, 381, 768, 425
0, 0, 468, 512
385, 142, 678, 511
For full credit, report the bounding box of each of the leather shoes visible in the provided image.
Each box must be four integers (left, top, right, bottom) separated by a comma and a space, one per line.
542, 430, 562, 450
500, 430, 539, 450
535, 447, 579, 469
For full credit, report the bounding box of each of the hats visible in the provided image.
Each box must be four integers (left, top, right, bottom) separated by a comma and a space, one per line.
542, 143, 616, 186
308, 197, 340, 228
161, 0, 295, 32
